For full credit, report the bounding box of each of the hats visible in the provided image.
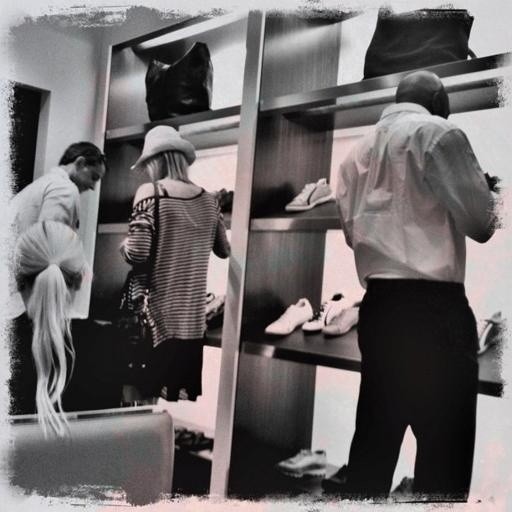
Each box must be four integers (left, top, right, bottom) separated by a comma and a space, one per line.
130, 123, 196, 170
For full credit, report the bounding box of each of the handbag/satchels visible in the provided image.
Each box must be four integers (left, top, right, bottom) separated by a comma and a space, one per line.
361, 6, 477, 78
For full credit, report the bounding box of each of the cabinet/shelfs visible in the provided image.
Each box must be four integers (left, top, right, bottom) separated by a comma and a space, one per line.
76, 4, 512, 502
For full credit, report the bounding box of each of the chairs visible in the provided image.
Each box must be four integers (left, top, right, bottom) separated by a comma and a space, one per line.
9, 404, 174, 506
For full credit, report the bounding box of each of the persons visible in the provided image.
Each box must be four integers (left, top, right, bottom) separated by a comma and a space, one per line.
3, 218, 122, 423
118, 125, 231, 415
334, 69, 498, 505
5, 141, 108, 321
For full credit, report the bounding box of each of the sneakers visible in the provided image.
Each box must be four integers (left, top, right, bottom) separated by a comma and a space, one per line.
284, 178, 335, 209
265, 297, 313, 338
279, 448, 327, 479
303, 293, 361, 336
478, 311, 505, 354
